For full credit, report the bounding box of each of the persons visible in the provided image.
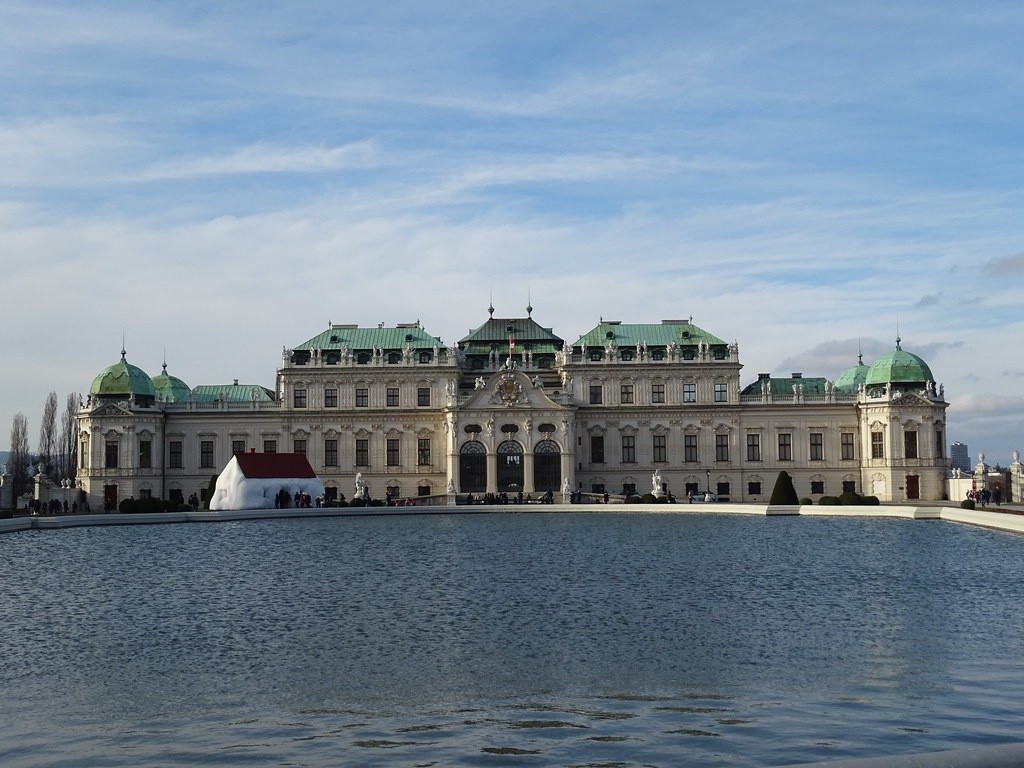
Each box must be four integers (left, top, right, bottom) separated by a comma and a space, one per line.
966, 486, 1006, 507
561, 477, 573, 504
447, 479, 456, 493
77, 338, 948, 409
29, 486, 695, 516
650, 469, 665, 499
354, 472, 366, 501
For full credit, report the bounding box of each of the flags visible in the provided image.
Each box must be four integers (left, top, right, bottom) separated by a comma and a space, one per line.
511, 338, 515, 352
